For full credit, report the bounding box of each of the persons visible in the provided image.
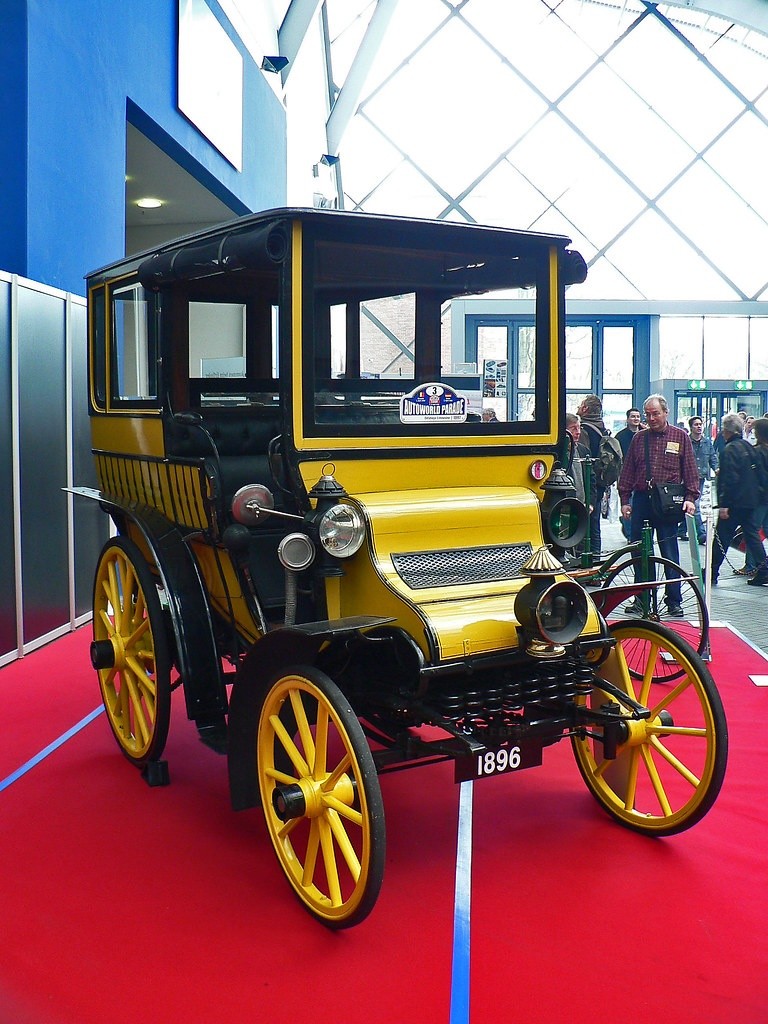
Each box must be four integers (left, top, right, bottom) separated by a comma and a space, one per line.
617, 394, 701, 618
565, 413, 598, 587
677, 416, 720, 543
482, 407, 499, 421
677, 408, 768, 509
576, 395, 608, 562
732, 417, 768, 575
558, 551, 576, 567
614, 407, 647, 543
701, 411, 768, 586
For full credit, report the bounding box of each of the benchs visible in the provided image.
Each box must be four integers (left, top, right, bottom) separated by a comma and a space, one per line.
169, 404, 403, 612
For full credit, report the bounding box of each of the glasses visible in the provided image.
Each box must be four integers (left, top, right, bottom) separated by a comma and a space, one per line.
644, 411, 663, 418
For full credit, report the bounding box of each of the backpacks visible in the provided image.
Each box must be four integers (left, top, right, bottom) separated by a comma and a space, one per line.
581, 423, 623, 490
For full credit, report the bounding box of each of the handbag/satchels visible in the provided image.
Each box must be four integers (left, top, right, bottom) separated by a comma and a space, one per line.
649, 484, 686, 521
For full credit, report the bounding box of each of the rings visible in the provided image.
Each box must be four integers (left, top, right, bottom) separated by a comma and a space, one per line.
691, 510, 692, 513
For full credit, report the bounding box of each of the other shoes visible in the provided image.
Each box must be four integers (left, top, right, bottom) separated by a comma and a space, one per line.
668, 605, 683, 616
625, 604, 642, 613
733, 565, 753, 575
747, 574, 768, 585
701, 568, 718, 584
698, 534, 707, 544
678, 534, 689, 541
